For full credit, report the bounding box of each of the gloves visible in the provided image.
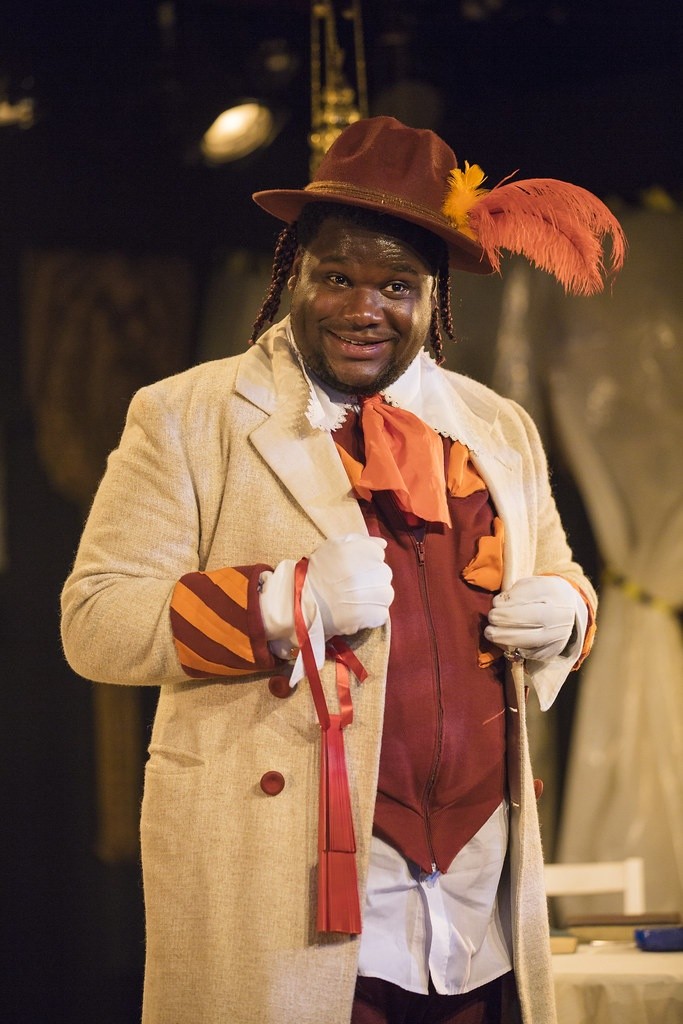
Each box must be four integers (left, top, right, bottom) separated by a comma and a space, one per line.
308, 534, 396, 641
484, 575, 576, 660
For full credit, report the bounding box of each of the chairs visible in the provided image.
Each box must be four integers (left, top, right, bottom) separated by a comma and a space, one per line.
542, 857, 648, 916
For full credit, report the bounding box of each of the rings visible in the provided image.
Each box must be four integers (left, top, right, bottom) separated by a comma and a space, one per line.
512, 646, 524, 661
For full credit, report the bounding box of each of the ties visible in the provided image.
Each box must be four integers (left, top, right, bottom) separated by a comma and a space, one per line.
356, 396, 453, 527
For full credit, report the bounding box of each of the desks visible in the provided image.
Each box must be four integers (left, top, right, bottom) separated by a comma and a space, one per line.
550, 938, 683, 1024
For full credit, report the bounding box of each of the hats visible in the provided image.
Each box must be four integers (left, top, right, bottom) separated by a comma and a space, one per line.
253, 116, 500, 277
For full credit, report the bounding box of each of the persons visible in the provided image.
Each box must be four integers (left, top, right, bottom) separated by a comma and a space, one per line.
59, 115, 627, 1024
